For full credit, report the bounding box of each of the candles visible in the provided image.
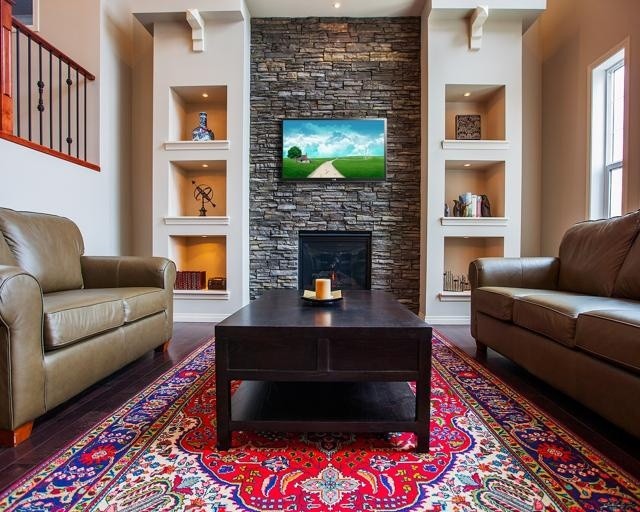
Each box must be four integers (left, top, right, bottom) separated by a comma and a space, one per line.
315, 278, 331, 300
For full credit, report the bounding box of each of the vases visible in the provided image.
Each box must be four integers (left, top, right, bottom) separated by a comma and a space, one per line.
191, 112, 214, 141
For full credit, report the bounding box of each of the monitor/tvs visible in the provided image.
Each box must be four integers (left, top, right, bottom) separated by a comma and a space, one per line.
279, 117, 387, 183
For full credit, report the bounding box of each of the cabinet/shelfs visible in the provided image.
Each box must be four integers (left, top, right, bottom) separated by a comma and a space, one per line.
152, 85, 250, 324
419, 84, 523, 326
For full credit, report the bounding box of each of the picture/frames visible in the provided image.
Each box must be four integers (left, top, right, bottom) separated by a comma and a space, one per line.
11, 0, 40, 32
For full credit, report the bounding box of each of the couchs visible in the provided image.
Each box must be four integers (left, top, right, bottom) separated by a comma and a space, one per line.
467, 210, 640, 441
0, 207, 177, 447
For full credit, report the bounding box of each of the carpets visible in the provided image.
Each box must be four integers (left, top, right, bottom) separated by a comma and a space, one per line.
0, 326, 640, 512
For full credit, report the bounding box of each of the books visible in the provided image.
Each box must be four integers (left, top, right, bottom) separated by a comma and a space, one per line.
173, 271, 207, 290
443, 269, 472, 292
459, 192, 483, 217
